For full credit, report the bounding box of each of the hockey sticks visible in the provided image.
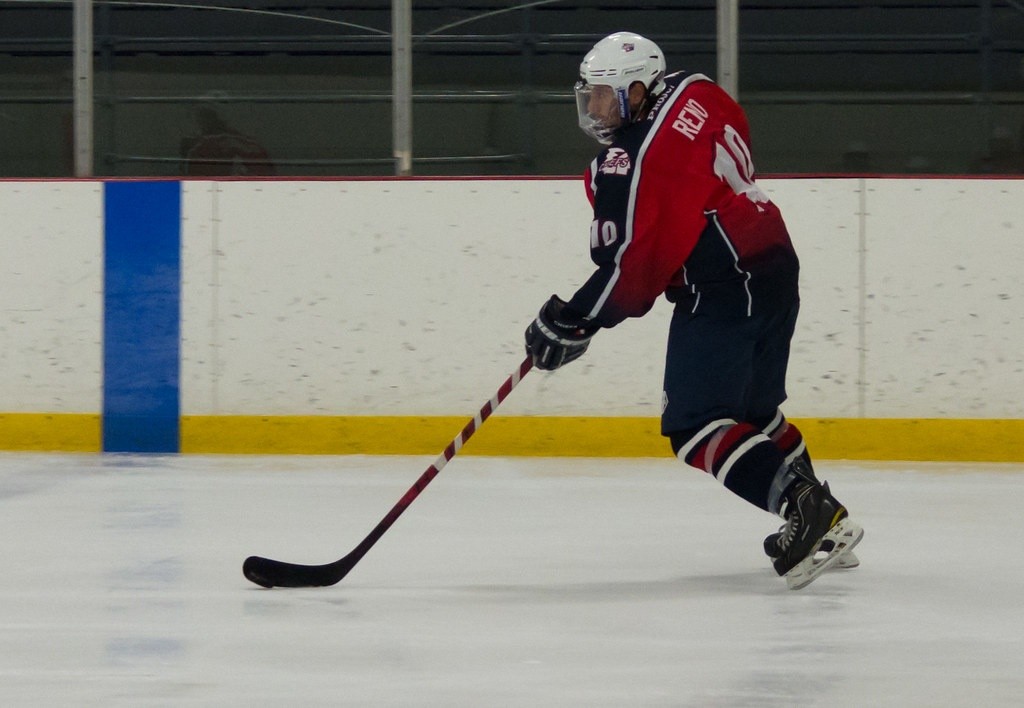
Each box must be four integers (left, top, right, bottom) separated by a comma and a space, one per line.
244, 355, 537, 590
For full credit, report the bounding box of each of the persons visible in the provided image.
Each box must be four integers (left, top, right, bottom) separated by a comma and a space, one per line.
526, 31, 865, 589
167, 88, 274, 175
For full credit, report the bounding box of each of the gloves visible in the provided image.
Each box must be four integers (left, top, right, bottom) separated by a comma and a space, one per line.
526, 294, 593, 370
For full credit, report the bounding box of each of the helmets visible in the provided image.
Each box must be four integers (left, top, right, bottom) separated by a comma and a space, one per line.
580, 32, 668, 99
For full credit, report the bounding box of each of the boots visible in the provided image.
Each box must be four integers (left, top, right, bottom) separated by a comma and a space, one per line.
773, 457, 865, 590
763, 480, 859, 567
